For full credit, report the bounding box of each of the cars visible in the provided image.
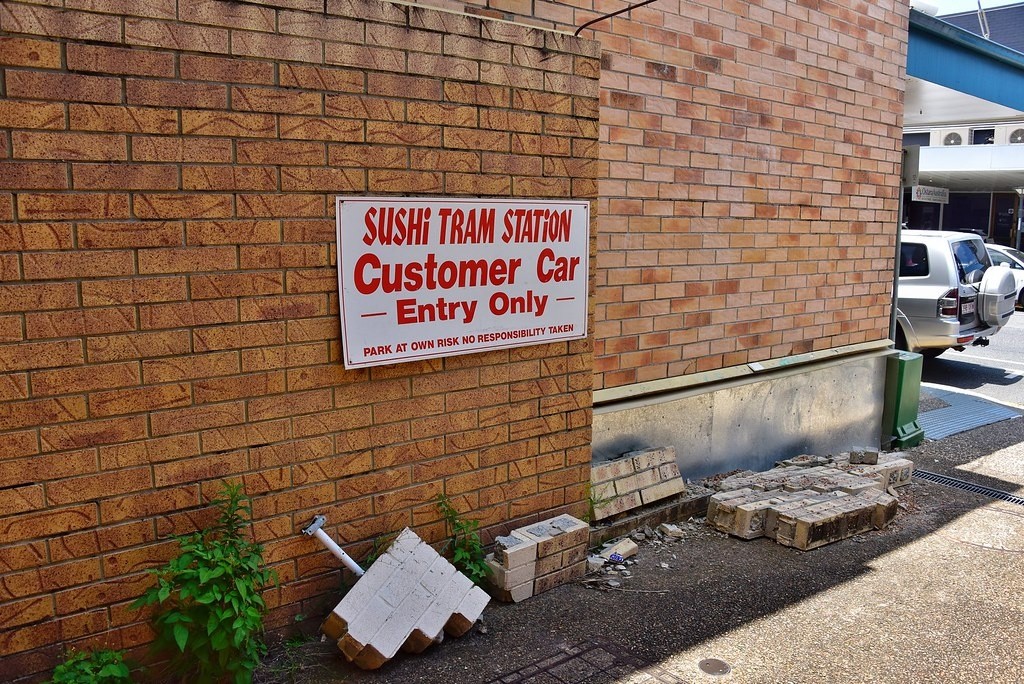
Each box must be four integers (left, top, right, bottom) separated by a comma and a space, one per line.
984, 242, 1024, 310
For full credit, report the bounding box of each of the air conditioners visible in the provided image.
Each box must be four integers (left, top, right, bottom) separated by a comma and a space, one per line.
1005, 124, 1024, 143
929, 127, 973, 145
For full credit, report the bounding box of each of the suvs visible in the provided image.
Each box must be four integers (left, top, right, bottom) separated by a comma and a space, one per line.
892, 229, 1016, 359
